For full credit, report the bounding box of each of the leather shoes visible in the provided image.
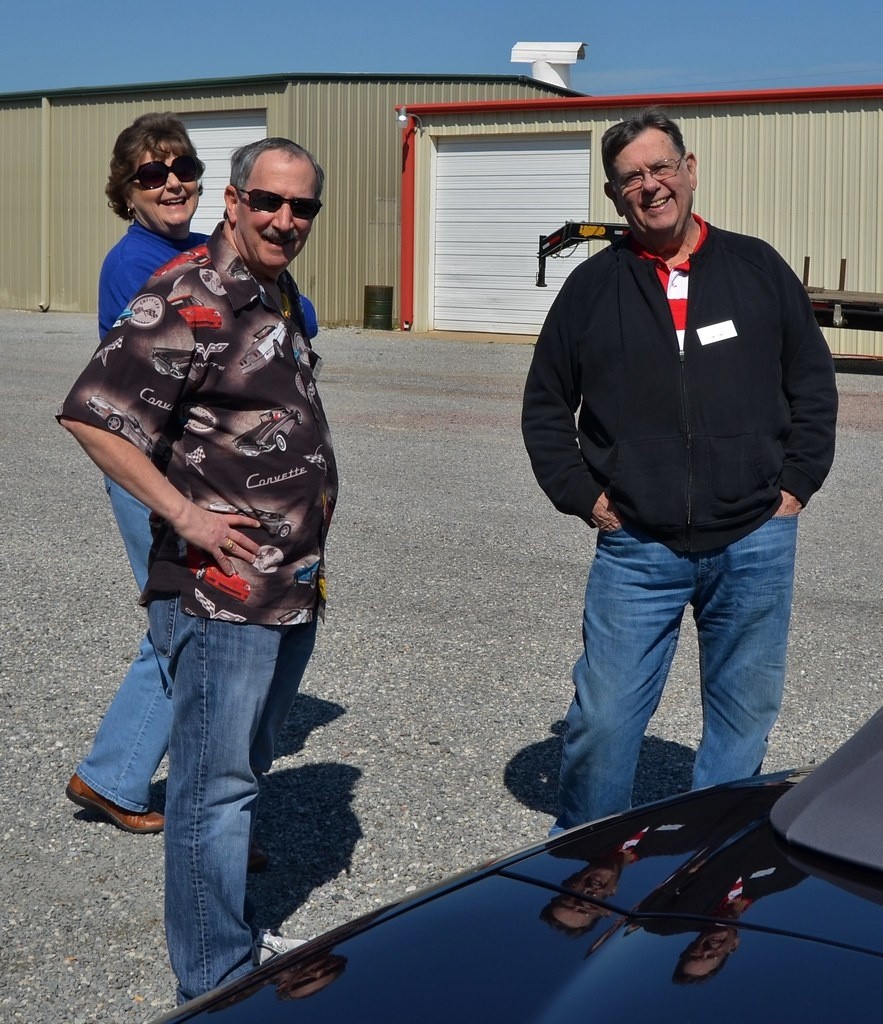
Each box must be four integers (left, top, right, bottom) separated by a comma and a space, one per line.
65, 772, 164, 833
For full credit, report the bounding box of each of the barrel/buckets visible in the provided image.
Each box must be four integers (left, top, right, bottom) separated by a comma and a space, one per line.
363, 285, 394, 331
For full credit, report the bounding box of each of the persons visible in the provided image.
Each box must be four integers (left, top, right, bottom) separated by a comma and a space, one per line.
519, 108, 834, 838
266, 916, 365, 1006
537, 775, 804, 983
54, 138, 337, 1010
63, 112, 318, 834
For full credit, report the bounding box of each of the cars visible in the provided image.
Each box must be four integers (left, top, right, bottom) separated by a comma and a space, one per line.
145, 706, 883, 1023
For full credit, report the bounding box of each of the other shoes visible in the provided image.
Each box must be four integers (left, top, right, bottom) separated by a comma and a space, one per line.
251, 928, 309, 966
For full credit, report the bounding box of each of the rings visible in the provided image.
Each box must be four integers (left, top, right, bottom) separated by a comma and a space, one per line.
226, 538, 234, 551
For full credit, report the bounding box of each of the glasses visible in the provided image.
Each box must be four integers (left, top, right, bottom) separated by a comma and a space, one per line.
232, 185, 323, 220
609, 153, 685, 195
687, 950, 725, 963
288, 964, 339, 993
559, 901, 594, 918
569, 878, 603, 900
126, 155, 198, 190
